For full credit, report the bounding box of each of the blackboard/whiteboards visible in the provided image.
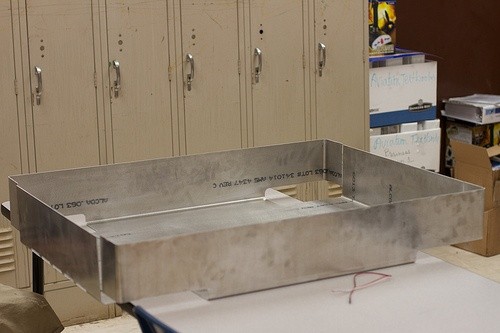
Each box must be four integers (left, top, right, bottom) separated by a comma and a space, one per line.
369, 60, 438, 114
370, 128, 442, 172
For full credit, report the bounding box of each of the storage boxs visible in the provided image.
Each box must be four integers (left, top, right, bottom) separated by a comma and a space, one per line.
368, 61, 437, 127
451, 180, 500, 257
451, 140, 500, 212
371, 128, 441, 173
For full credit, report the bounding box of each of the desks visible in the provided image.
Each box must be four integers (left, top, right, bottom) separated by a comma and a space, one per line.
2, 188, 500, 333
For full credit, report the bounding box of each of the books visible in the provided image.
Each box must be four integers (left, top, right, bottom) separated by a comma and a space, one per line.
439, 93, 500, 124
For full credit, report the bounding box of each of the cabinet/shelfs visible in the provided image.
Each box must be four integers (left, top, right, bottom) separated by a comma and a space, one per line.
181, 0, 242, 159
249, 0, 306, 148
313, 0, 364, 151
25, 0, 100, 174
0, 0, 31, 288
106, 0, 173, 164
